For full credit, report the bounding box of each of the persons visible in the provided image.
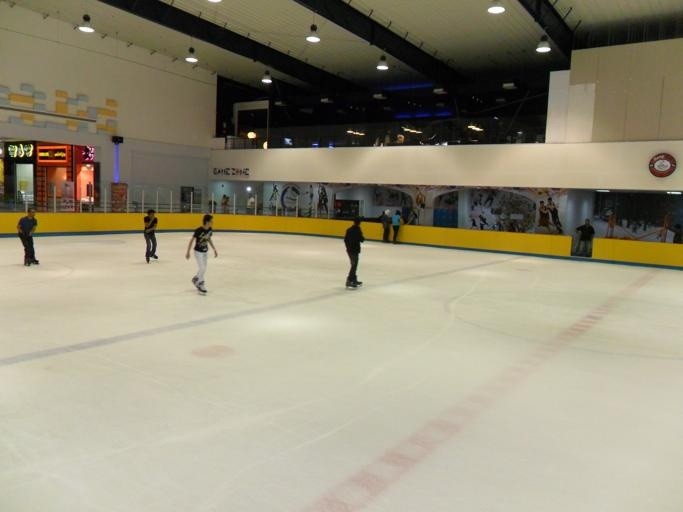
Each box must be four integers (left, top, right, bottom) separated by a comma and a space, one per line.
247, 193, 255, 214
143, 209, 159, 263
372, 126, 406, 146
185, 214, 219, 293
414, 190, 426, 208
380, 208, 392, 242
405, 210, 417, 225
343, 216, 365, 289
269, 184, 280, 210
221, 194, 229, 214
17, 207, 39, 266
317, 184, 328, 215
307, 185, 314, 205
391, 210, 401, 244
470, 190, 649, 241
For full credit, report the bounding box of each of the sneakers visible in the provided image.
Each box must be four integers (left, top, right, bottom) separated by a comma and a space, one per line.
25, 260, 30, 266
150, 252, 158, 259
31, 260, 39, 264
146, 255, 149, 263
346, 281, 357, 287
355, 282, 363, 285
196, 281, 207, 292
192, 278, 199, 286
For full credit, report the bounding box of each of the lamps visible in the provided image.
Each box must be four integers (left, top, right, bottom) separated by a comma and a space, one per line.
376, 48, 389, 71
488, 0, 506, 15
78, 14, 94, 32
184, 37, 199, 62
307, 9, 320, 43
535, 35, 551, 52
